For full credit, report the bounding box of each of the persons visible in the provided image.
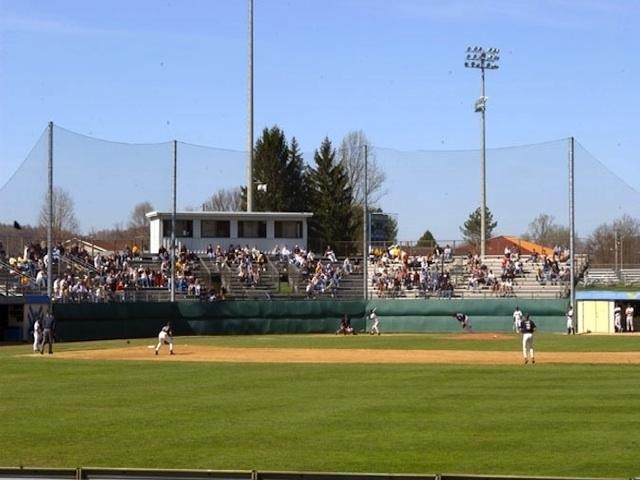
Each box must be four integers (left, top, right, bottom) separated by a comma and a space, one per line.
343, 257, 361, 275
154, 322, 176, 356
291, 244, 342, 298
614, 303, 624, 332
159, 244, 224, 301
52, 240, 164, 302
227, 244, 266, 288
625, 304, 634, 330
511, 305, 522, 333
369, 244, 457, 300
520, 313, 538, 366
336, 319, 355, 335
486, 269, 516, 297
272, 243, 294, 267
452, 312, 472, 330
466, 251, 488, 288
525, 243, 572, 286
32, 317, 42, 353
501, 244, 523, 282
40, 311, 57, 355
9, 241, 48, 287
565, 307, 575, 335
367, 310, 380, 335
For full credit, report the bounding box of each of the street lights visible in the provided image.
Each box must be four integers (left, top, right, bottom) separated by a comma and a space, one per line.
464, 44, 501, 264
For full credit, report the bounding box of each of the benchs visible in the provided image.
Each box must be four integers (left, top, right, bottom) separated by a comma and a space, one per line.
584, 266, 640, 288
0, 253, 590, 300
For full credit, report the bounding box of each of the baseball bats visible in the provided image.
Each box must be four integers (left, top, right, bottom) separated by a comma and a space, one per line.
371, 308, 377, 314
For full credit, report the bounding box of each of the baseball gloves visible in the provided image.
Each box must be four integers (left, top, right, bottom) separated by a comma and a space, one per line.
164, 341, 168, 344
